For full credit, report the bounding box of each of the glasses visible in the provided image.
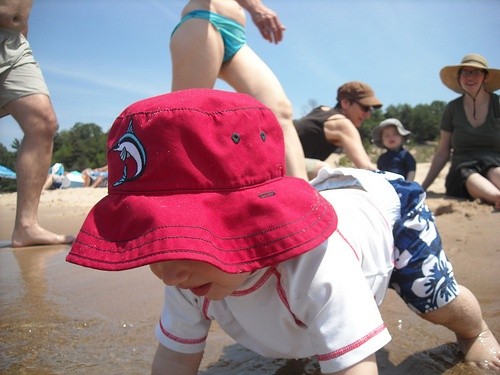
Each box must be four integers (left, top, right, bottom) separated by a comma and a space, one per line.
359, 105, 373, 113
461, 69, 484, 76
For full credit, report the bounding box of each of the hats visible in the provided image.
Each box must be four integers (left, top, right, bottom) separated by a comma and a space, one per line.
65, 89, 338, 274
438, 53, 500, 94
336, 81, 383, 111
372, 118, 411, 150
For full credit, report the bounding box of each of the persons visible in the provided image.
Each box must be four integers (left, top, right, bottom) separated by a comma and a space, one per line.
169, 0, 311, 182
85, 165, 108, 188
65, 88, 500, 375
372, 118, 416, 182
0, 0, 76, 247
420, 54, 500, 210
295, 82, 380, 180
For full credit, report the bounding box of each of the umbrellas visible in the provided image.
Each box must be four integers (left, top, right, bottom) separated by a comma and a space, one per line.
0, 165, 16, 180
